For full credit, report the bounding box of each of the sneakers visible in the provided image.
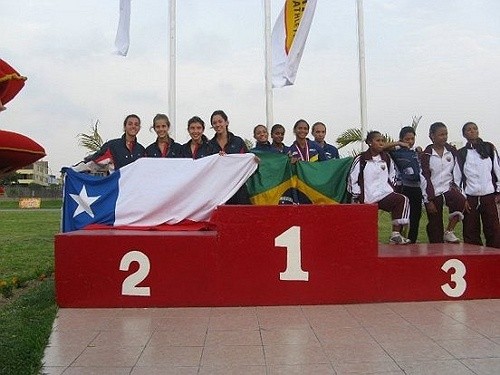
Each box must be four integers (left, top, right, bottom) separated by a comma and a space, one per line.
389, 233, 411, 245
443, 231, 460, 243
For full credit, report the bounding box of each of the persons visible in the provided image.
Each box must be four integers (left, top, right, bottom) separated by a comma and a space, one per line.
61, 110, 499, 249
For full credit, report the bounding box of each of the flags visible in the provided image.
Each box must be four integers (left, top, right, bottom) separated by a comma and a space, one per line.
114, 0, 130, 56
269, 0, 316, 87
243, 150, 354, 205
62, 153, 258, 234
